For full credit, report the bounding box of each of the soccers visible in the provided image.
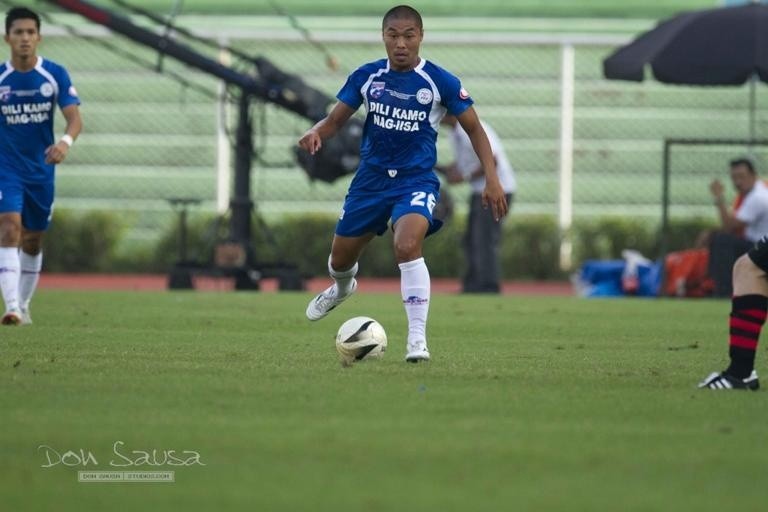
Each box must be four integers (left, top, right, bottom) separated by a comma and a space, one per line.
335, 316, 389, 367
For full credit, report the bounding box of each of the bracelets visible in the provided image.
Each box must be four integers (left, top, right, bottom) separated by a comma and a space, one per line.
60, 134, 75, 147
714, 195, 726, 205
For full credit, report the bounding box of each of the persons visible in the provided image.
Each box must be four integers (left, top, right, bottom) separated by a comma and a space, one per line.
295, 4, 509, 368
432, 110, 516, 292
696, 156, 768, 298
0, 5, 85, 327
697, 234, 768, 389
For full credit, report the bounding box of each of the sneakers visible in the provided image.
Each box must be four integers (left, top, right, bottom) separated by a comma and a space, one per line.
406, 338, 431, 361
2, 309, 33, 327
306, 278, 358, 322
698, 370, 761, 392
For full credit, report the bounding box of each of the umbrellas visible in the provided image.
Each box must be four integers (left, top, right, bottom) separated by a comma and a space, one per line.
601, 0, 767, 141
